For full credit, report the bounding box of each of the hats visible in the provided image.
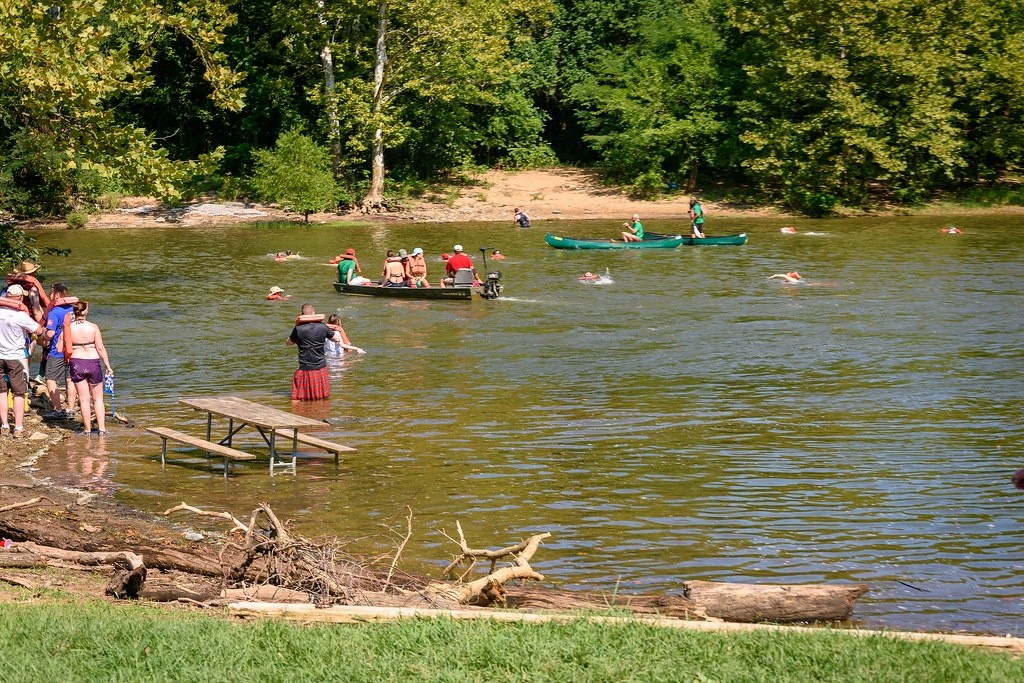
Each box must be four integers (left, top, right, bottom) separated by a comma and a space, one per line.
347, 248, 355, 256
269, 286, 284, 296
454, 245, 463, 251
13, 259, 41, 274
6, 284, 28, 296
411, 248, 423, 256
398, 249, 407, 258
690, 197, 697, 201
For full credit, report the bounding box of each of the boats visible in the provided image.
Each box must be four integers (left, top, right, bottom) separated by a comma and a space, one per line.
642, 230, 748, 246
330, 267, 504, 301
544, 231, 684, 250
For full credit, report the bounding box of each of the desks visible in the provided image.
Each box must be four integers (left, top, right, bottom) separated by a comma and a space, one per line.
180, 395, 330, 477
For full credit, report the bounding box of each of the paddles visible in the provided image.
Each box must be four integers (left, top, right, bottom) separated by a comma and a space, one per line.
688, 210, 701, 236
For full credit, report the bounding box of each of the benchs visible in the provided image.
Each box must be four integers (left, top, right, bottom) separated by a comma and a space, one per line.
266, 428, 358, 464
145, 426, 257, 479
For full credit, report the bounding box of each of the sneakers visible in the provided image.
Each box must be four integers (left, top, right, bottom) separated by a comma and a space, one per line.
0, 427, 10, 436
35, 374, 47, 387
13, 427, 29, 437
66, 411, 74, 418
42, 409, 66, 420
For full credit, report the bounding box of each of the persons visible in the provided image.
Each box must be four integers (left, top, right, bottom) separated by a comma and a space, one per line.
335, 248, 372, 286
515, 208, 530, 227
378, 247, 431, 288
275, 250, 300, 261
769, 272, 801, 282
286, 304, 340, 401
780, 226, 799, 234
578, 272, 603, 280
610, 213, 644, 242
942, 226, 962, 233
689, 197, 705, 238
324, 314, 367, 354
491, 250, 505, 259
0, 262, 114, 439
440, 245, 483, 288
265, 286, 290, 300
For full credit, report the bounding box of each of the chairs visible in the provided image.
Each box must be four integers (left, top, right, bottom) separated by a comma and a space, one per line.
453, 268, 475, 288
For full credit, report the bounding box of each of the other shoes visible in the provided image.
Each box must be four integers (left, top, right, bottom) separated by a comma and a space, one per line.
79, 431, 92, 435
97, 430, 110, 436
